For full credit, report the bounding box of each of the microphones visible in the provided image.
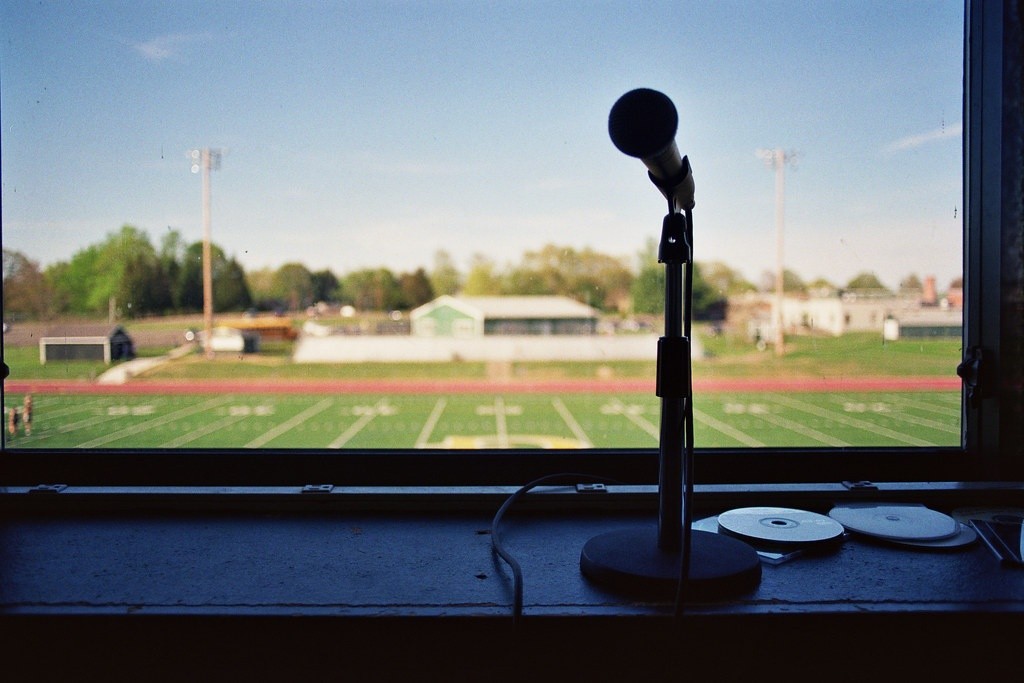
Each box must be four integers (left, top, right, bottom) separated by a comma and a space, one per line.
608, 87, 695, 210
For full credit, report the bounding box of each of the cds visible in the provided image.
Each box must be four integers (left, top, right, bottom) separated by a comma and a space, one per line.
716, 501, 978, 552
